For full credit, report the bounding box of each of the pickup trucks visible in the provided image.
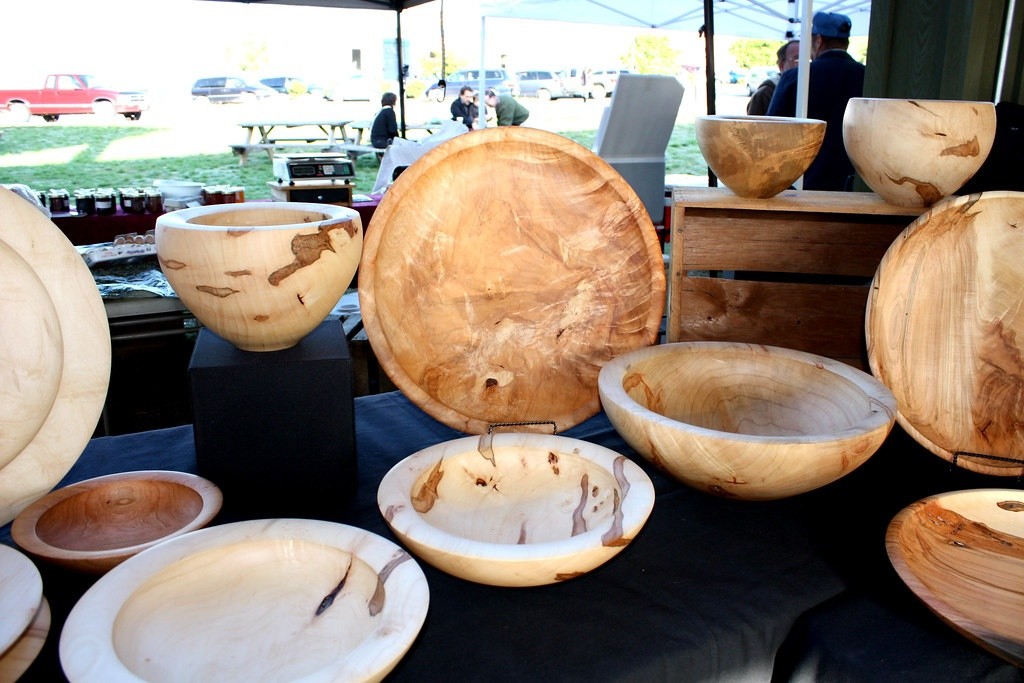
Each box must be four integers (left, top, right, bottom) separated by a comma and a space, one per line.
0, 69, 150, 123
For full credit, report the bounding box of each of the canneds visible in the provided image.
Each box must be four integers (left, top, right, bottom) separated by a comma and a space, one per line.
35, 186, 163, 216
202, 185, 244, 204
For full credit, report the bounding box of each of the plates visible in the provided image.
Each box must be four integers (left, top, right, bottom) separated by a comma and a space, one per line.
356, 126, 665, 433
886, 486, 1024, 670
0, 181, 111, 529
58, 517, 430, 682
865, 191, 1023, 477
0, 544, 51, 683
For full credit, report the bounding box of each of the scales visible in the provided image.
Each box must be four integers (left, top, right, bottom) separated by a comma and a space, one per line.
268, 136, 356, 204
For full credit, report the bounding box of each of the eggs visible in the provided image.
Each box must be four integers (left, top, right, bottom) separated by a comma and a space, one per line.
113, 235, 154, 246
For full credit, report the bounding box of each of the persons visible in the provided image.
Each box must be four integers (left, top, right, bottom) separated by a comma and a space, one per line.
450, 86, 492, 132
485, 90, 530, 127
368, 93, 401, 159
746, 39, 799, 116
766, 11, 866, 190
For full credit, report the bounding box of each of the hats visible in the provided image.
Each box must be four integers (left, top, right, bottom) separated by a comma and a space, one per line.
811, 12, 852, 38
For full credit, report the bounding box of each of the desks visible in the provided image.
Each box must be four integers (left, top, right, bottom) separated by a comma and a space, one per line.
236, 118, 357, 168
0, 387, 854, 683
353, 118, 443, 166
40, 193, 387, 290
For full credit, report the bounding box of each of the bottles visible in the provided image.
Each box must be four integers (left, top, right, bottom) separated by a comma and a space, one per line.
35, 184, 245, 216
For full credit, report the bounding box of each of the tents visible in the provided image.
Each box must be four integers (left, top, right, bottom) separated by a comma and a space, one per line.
476, 0, 869, 189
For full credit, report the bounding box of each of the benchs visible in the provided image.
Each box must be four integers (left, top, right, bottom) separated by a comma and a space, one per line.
227, 136, 418, 158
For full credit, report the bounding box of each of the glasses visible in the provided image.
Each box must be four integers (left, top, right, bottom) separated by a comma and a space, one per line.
785, 57, 813, 65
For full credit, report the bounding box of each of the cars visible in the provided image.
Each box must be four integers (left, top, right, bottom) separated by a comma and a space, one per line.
257, 76, 301, 94
510, 69, 570, 100
191, 75, 271, 105
425, 67, 521, 106
553, 66, 633, 101
728, 64, 781, 97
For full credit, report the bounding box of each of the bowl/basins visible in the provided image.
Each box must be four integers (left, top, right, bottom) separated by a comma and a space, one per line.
696, 111, 829, 200
151, 200, 365, 353
374, 432, 657, 589
599, 341, 899, 504
842, 97, 997, 209
12, 470, 223, 572
155, 183, 206, 198
